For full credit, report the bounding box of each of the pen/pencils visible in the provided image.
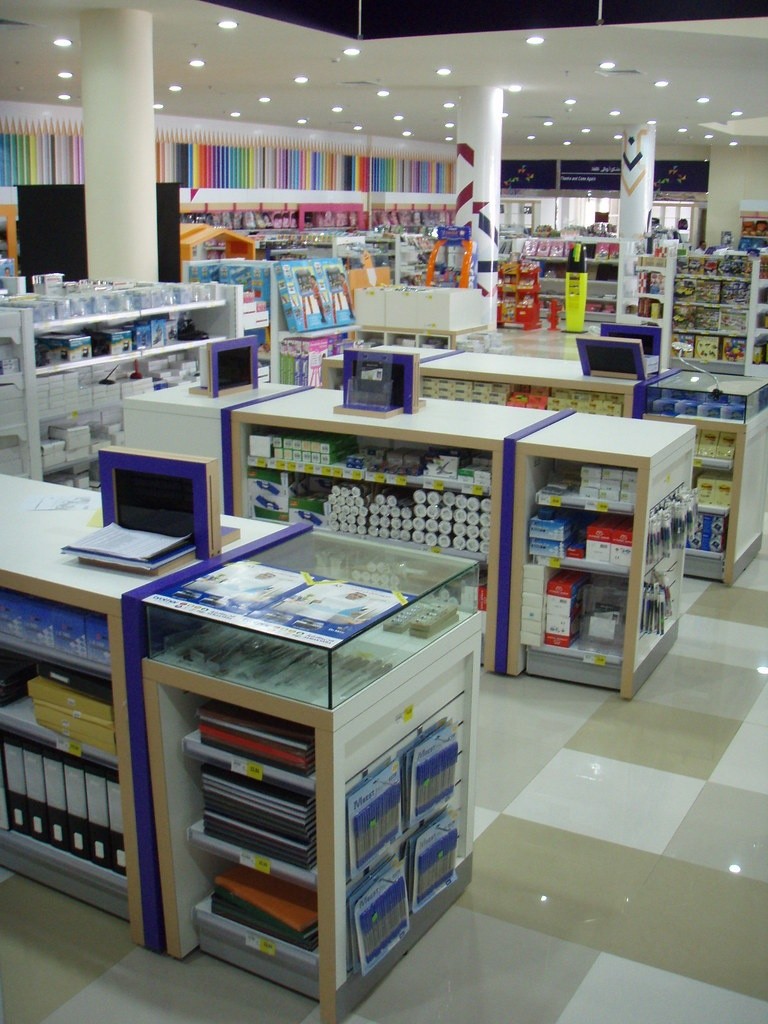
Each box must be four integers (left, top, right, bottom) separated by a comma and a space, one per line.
353, 739, 458, 963
641, 577, 673, 635
647, 488, 698, 564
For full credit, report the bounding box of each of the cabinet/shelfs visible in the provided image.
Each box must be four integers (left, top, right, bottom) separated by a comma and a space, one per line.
501, 238, 618, 324
360, 234, 437, 287
120, 382, 695, 701
0, 468, 478, 1024
321, 345, 768, 586
181, 257, 359, 385
630, 250, 768, 378
0, 282, 243, 481
258, 236, 366, 262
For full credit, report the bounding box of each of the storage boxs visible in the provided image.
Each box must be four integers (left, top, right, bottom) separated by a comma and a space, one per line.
0, 316, 197, 474
674, 257, 753, 363
0, 591, 129, 877
418, 374, 626, 416
520, 465, 637, 647
188, 257, 357, 383
645, 385, 746, 550
